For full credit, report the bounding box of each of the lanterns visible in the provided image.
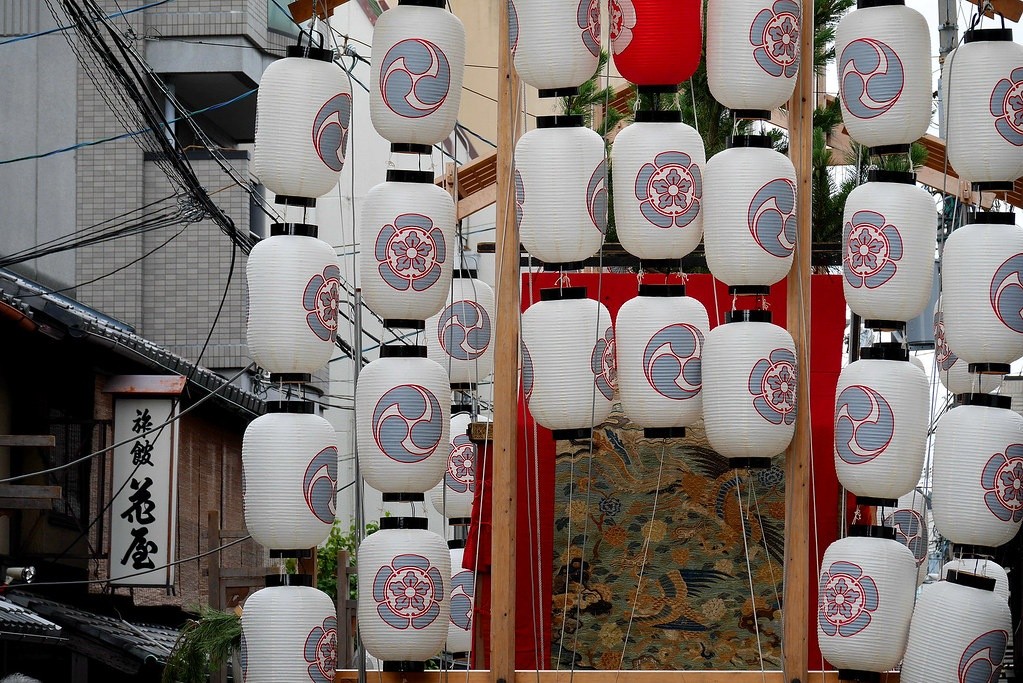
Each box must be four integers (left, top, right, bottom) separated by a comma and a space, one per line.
239, 0, 1023, 683
